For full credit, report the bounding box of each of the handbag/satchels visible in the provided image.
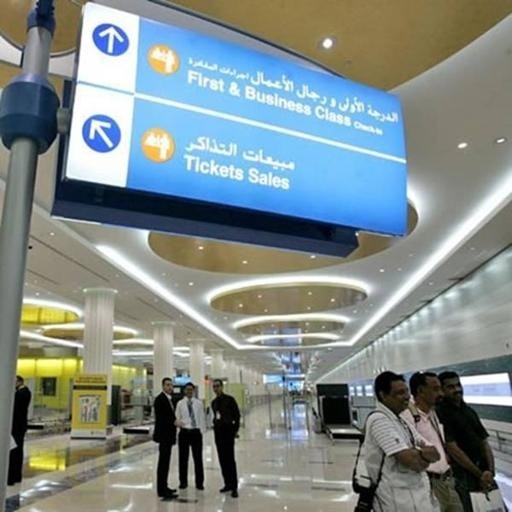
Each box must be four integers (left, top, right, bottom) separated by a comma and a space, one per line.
468, 481, 506, 512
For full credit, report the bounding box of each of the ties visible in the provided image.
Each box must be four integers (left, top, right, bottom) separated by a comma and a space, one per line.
187, 399, 196, 429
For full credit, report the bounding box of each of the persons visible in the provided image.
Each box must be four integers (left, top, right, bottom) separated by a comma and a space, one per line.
152, 377, 184, 499
363, 371, 442, 512
437, 371, 509, 512
175, 382, 206, 490
400, 370, 465, 511
7, 374, 31, 485
211, 379, 241, 497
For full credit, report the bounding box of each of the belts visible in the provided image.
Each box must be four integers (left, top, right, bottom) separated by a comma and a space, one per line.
426, 469, 452, 482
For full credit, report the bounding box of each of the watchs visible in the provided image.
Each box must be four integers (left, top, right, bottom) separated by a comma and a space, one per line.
415, 444, 423, 454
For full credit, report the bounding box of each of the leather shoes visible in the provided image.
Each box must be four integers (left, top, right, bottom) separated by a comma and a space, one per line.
197, 485, 203, 490
232, 490, 238, 498
179, 484, 188, 488
168, 488, 176, 493
219, 486, 230, 493
164, 493, 179, 500
